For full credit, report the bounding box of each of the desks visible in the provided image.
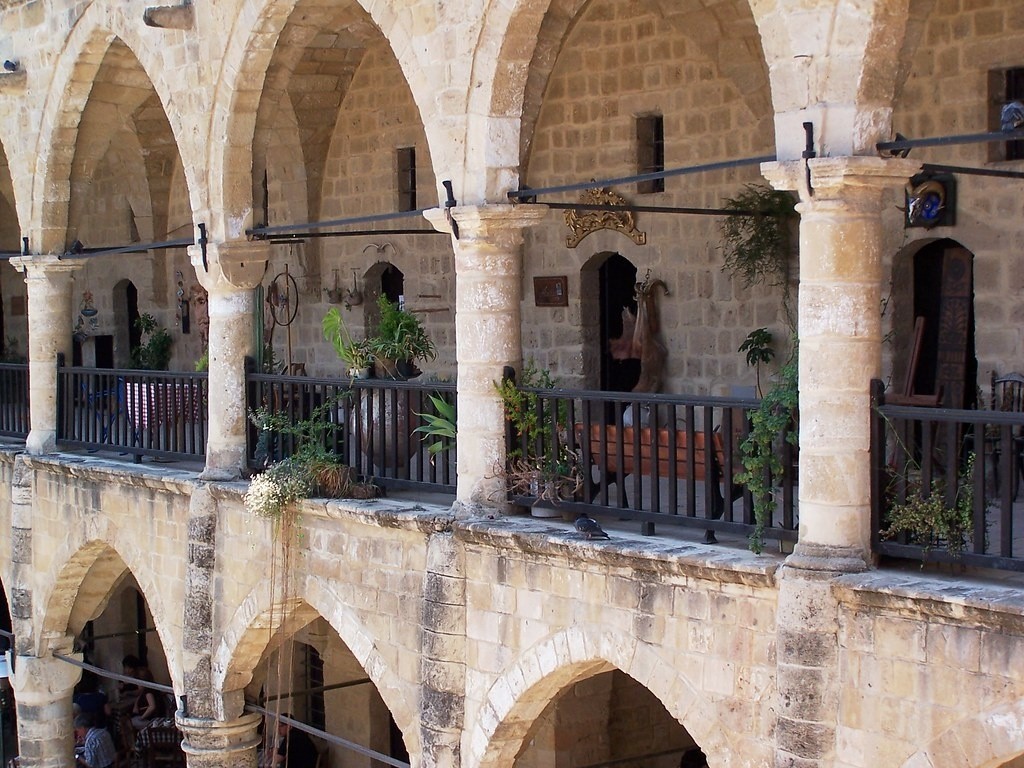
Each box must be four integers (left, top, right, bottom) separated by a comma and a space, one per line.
125, 382, 203, 463
135, 718, 183, 761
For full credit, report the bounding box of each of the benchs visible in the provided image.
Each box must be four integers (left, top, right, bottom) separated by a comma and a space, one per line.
567, 422, 757, 545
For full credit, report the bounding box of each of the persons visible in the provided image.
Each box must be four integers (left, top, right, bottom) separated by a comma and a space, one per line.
72, 637, 164, 768
269, 713, 318, 768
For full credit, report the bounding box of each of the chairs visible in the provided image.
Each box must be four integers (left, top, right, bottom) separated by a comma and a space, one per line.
118, 693, 186, 768
965, 372, 1024, 501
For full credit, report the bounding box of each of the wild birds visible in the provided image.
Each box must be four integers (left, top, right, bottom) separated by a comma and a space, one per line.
574, 518, 610, 541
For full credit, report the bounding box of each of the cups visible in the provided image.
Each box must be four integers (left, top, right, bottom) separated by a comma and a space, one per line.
153, 721, 158, 727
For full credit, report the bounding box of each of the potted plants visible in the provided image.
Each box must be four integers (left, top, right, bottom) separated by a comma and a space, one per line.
321, 308, 372, 380
0, 336, 27, 403
373, 295, 438, 379
123, 313, 200, 428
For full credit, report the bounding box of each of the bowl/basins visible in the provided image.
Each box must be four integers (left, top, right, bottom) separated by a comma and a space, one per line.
75, 747, 85, 755
163, 721, 173, 728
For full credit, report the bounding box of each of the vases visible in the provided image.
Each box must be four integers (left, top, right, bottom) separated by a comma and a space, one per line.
528, 481, 574, 518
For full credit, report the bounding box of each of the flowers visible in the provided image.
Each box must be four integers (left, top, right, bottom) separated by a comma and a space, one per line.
491, 355, 582, 481
